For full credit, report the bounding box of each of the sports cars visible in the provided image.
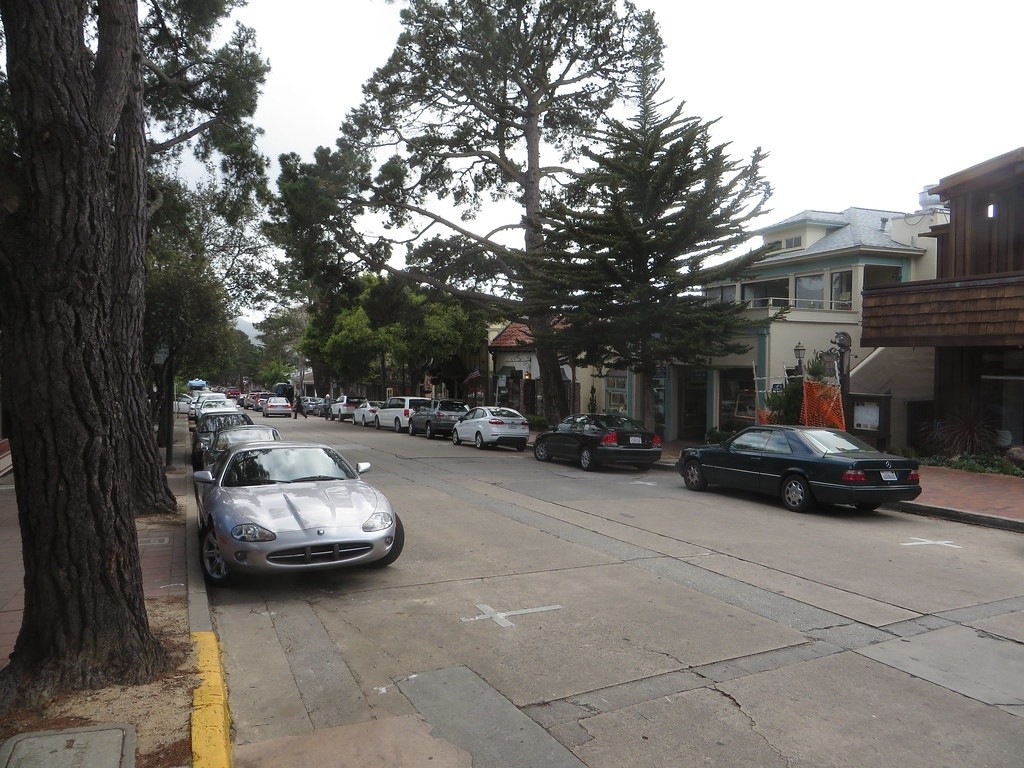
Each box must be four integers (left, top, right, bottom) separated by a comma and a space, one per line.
192, 440, 405, 586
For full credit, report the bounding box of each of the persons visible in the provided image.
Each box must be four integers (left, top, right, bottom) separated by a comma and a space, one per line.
293, 394, 332, 421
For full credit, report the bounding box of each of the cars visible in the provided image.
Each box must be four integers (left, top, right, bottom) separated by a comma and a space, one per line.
677, 425, 924, 515
197, 399, 239, 420
353, 400, 385, 426
201, 425, 284, 476
188, 411, 257, 466
146, 392, 193, 414
451, 405, 530, 451
533, 411, 663, 471
263, 396, 292, 418
190, 378, 334, 418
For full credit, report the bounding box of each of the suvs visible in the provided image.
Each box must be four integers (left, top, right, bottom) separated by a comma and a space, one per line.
328, 395, 369, 422
408, 397, 470, 439
373, 396, 431, 433
